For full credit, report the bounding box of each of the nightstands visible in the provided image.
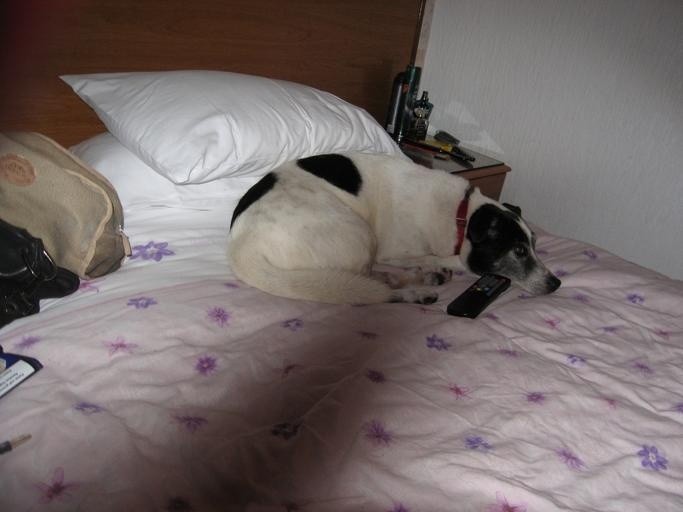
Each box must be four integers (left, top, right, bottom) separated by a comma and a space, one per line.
394, 130, 512, 201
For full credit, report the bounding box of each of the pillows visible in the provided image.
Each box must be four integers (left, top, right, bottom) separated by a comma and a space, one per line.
66, 131, 276, 225
59, 69, 412, 184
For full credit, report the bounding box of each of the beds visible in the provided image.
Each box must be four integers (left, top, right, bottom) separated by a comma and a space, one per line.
1, 0, 683, 512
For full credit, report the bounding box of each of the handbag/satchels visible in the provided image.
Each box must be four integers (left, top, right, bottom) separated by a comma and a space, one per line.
0, 130, 124, 279
0, 218, 79, 320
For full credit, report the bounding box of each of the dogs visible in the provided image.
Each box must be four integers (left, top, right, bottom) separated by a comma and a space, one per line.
226, 149, 562, 307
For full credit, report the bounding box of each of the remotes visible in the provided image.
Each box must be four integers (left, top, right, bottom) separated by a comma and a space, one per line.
449, 273, 512, 318
